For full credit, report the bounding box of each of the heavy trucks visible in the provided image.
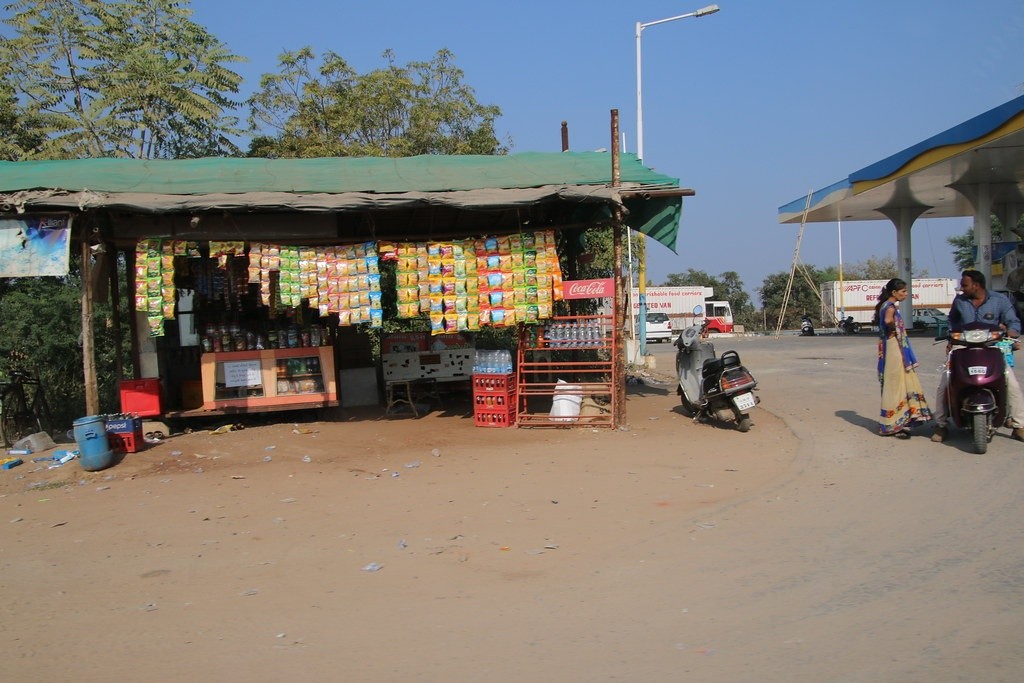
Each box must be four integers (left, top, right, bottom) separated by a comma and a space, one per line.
624, 287, 733, 334
820, 278, 964, 328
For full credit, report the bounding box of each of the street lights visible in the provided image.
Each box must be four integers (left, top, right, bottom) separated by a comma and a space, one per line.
635, 3, 720, 365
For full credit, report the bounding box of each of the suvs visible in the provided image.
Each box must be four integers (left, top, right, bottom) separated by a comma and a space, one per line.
635, 312, 672, 343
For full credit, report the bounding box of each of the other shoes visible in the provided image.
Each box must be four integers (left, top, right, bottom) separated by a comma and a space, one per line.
903, 419, 924, 427
931, 427, 948, 441
1012, 428, 1024, 441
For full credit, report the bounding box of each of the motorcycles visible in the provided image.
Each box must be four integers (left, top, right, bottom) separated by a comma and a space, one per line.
840, 315, 862, 334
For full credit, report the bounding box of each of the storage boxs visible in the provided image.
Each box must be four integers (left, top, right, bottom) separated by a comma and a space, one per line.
104, 419, 144, 454
119, 377, 162, 419
473, 372, 517, 428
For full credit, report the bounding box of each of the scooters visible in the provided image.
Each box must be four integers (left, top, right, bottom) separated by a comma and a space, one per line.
673, 304, 760, 432
800, 314, 814, 336
932, 313, 1006, 454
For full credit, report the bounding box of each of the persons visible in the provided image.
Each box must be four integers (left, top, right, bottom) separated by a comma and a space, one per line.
932, 270, 1024, 443
872, 278, 933, 439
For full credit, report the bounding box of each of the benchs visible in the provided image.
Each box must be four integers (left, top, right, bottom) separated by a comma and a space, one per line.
385, 377, 445, 419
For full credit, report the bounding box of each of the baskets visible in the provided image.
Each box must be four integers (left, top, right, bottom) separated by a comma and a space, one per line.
108, 430, 144, 453
472, 373, 517, 427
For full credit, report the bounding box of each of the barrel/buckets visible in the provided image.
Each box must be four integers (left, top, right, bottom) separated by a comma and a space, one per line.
72, 415, 114, 471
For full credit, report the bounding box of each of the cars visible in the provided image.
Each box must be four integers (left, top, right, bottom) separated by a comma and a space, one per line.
912, 308, 949, 328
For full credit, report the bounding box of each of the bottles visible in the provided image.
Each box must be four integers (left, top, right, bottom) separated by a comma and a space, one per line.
275, 359, 319, 376
101, 412, 141, 445
536, 321, 600, 348
473, 350, 513, 422
